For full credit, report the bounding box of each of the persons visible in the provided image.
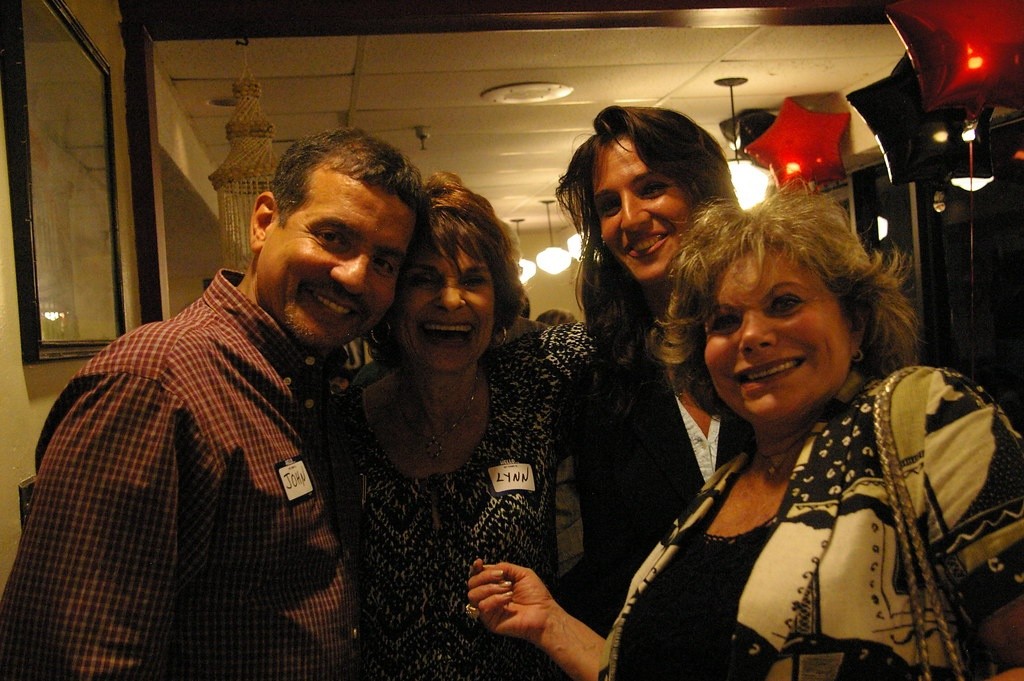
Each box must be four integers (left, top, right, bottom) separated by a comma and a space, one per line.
0, 129, 427, 681
386, 170, 673, 676
560, 108, 755, 680
462, 184, 1023, 679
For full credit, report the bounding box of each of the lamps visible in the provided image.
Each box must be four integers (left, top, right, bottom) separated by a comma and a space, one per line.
714, 78, 768, 210
510, 219, 536, 284
536, 201, 571, 274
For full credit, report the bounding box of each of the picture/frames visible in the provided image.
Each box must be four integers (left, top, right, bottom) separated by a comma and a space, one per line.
0, 0, 144, 365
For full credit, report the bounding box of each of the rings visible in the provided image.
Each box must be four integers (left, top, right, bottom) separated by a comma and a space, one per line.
465, 602, 480, 621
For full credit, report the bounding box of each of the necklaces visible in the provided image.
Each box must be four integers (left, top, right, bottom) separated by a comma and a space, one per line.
392, 366, 478, 466
760, 428, 814, 482
